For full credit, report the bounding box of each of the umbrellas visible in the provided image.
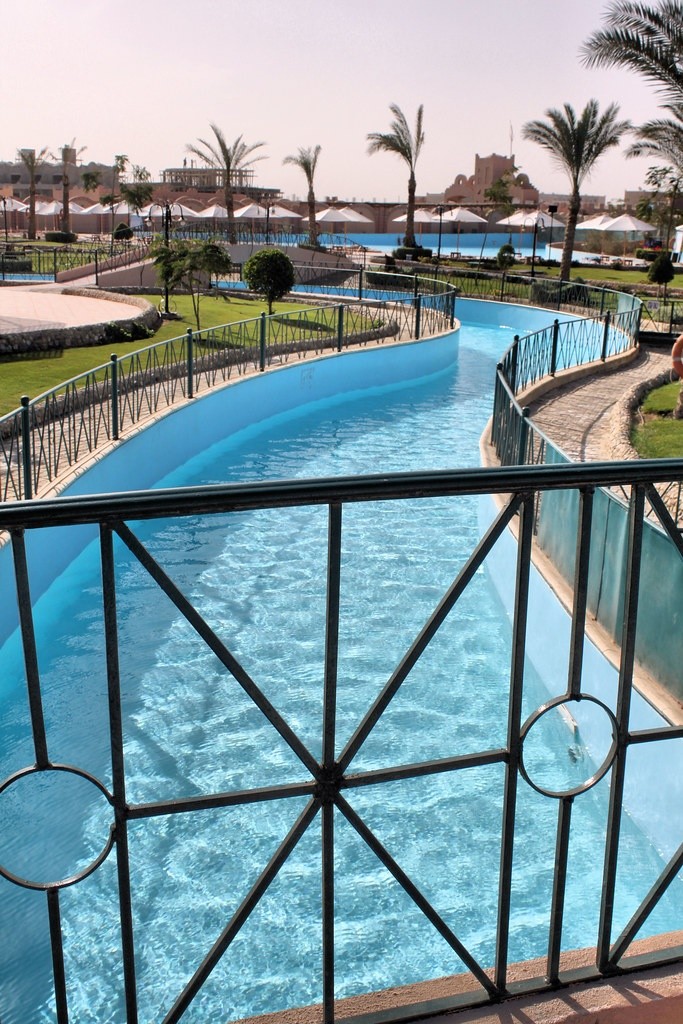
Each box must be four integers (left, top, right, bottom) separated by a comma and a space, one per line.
0, 198, 374, 245
392, 207, 488, 253
496, 210, 658, 259
